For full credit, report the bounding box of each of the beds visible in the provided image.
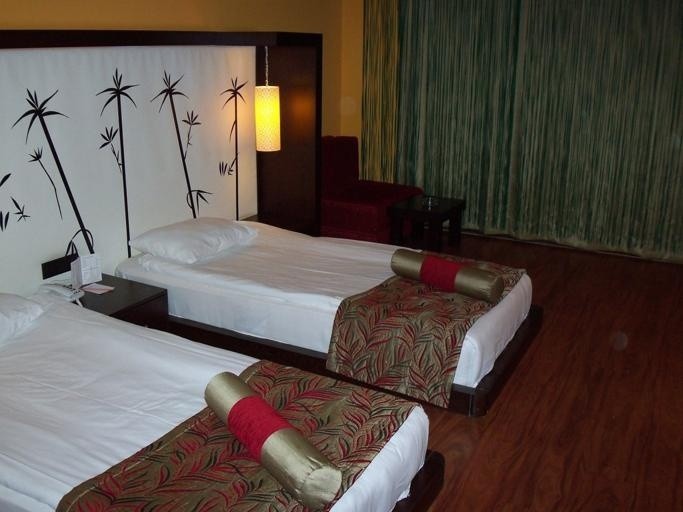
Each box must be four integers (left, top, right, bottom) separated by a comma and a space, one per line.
106, 220, 546, 421
0, 292, 449, 511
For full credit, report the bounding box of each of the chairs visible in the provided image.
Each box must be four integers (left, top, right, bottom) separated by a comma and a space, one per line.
321, 134, 423, 245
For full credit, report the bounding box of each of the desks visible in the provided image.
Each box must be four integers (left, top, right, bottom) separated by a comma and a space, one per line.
387, 193, 468, 252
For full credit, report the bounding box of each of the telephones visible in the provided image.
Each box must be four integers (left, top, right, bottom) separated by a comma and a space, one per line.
42, 283, 85, 303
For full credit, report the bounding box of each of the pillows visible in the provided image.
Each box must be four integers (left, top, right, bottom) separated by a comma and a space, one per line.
129, 219, 260, 271
1, 289, 44, 350
390, 247, 506, 305
203, 370, 346, 512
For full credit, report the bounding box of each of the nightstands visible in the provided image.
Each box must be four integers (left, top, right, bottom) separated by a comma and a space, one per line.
65, 274, 173, 334
248, 215, 315, 239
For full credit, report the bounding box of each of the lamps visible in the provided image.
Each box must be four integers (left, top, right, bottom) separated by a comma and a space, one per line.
253, 39, 283, 156
